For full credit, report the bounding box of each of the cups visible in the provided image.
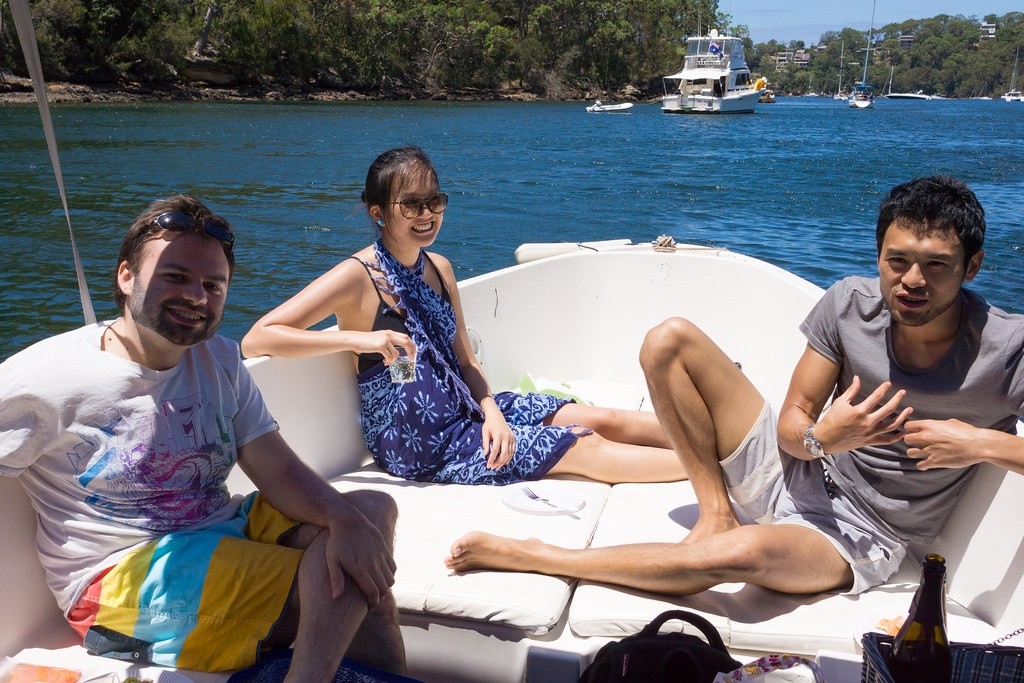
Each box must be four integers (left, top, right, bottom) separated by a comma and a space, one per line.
389, 346, 416, 383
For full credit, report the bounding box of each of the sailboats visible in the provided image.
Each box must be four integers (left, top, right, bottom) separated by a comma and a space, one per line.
833, 40, 848, 102
999, 46, 1024, 102
847, 0, 878, 110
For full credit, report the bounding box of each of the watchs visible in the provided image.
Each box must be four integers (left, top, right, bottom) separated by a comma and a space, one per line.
803, 424, 829, 458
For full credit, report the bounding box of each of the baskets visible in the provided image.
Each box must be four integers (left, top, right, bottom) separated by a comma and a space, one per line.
861, 632, 1024, 683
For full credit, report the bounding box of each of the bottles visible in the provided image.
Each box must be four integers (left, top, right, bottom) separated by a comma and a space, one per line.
890, 553, 951, 683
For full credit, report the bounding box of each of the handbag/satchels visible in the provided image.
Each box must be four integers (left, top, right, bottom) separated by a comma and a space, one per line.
579, 610, 742, 683
713, 653, 827, 683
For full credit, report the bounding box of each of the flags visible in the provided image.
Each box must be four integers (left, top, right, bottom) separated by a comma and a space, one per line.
708, 40, 723, 60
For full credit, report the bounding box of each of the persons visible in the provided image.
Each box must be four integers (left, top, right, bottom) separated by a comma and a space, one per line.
0, 195, 406, 683
444, 175, 1024, 595
241, 145, 693, 482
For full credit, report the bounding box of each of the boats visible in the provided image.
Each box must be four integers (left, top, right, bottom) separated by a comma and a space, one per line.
885, 91, 934, 100
968, 82, 994, 100
585, 101, 634, 112
658, 28, 767, 114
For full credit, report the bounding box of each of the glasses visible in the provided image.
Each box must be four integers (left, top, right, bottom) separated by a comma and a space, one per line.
139, 211, 234, 253
384, 192, 448, 219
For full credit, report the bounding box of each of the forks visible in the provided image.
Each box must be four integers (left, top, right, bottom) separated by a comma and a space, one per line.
521, 487, 581, 520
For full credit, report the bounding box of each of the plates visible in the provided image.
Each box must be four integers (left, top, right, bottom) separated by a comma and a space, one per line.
503, 484, 585, 513
